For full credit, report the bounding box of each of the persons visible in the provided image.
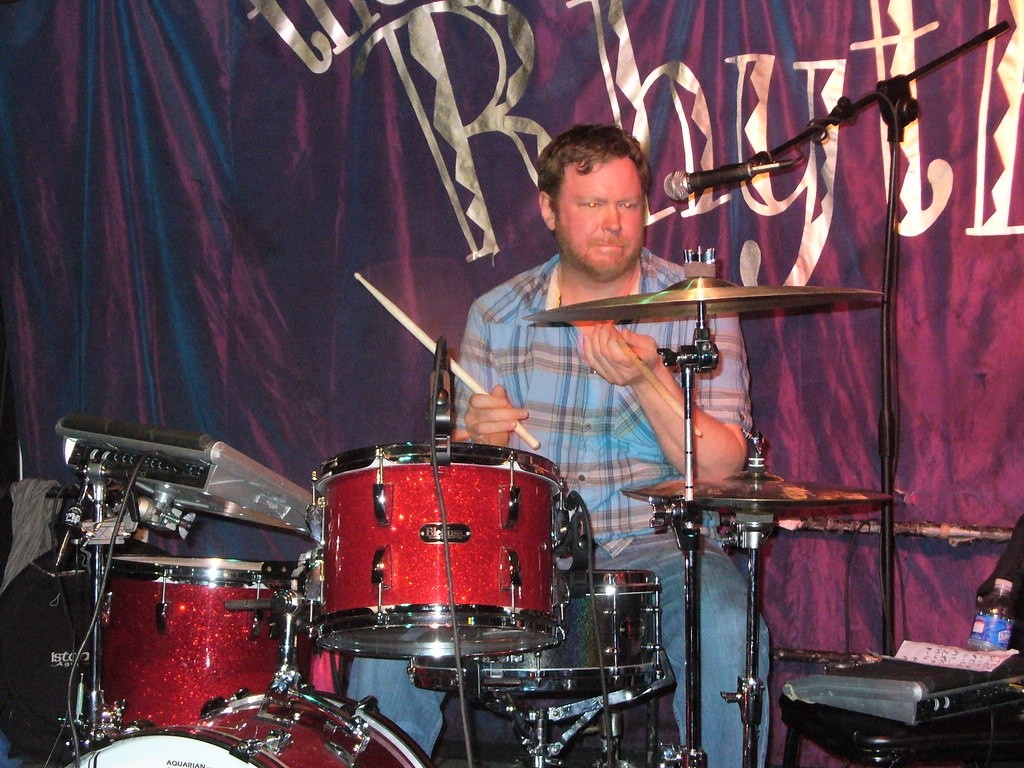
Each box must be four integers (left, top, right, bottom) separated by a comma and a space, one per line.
345, 124, 771, 768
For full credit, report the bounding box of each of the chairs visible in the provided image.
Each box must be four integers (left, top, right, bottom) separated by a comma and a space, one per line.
782, 515, 1024, 768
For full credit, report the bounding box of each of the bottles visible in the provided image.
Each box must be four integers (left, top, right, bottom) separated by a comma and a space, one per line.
967, 578, 1014, 651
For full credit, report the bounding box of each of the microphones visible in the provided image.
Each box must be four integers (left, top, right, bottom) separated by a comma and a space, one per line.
664, 160, 793, 201
427, 337, 456, 433
555, 490, 579, 560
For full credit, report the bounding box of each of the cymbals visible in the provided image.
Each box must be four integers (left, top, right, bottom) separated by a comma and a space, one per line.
521, 276, 886, 323
620, 467, 893, 515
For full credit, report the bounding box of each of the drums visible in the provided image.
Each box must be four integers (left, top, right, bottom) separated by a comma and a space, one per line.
57, 687, 436, 768
407, 569, 664, 696
93, 551, 309, 738
304, 438, 566, 660
